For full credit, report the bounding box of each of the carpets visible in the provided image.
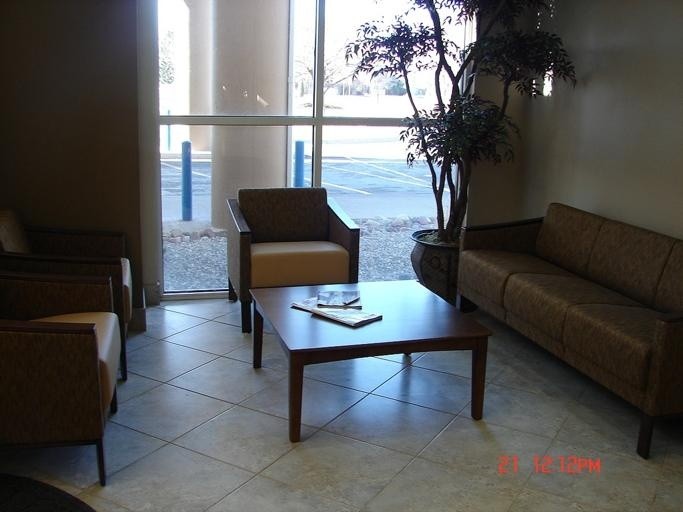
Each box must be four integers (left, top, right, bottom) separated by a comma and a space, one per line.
0, 471, 98, 511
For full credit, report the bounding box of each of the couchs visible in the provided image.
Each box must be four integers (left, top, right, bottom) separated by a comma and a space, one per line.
455, 201, 681, 461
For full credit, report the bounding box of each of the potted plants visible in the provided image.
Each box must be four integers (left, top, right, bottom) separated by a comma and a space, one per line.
344, 0, 577, 300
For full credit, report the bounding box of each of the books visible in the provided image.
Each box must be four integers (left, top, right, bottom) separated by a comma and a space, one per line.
292, 297, 382, 328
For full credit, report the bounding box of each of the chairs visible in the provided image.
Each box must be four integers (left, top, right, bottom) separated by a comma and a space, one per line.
0, 246, 124, 488
1, 199, 133, 384
226, 187, 359, 333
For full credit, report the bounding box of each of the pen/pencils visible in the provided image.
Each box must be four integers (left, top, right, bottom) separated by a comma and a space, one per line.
344, 296, 360, 306
